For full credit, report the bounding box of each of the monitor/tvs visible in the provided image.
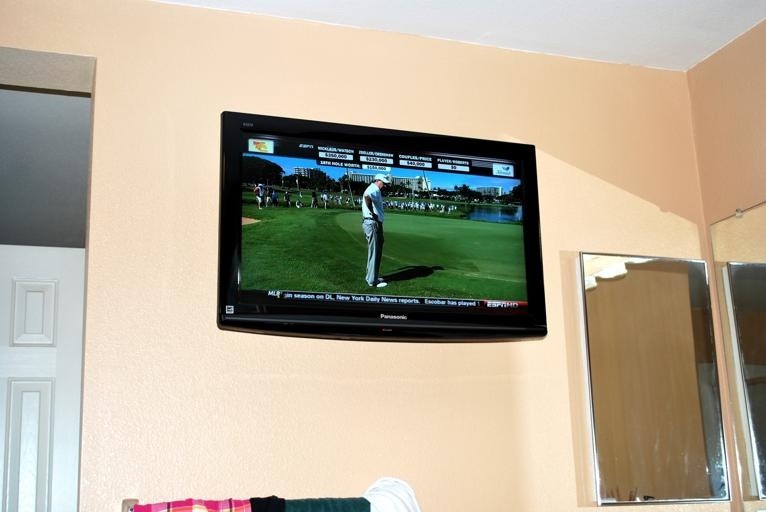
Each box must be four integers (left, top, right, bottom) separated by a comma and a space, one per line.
217, 111, 547, 342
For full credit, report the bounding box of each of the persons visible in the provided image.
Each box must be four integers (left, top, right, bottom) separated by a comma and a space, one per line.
329, 193, 458, 214
361, 172, 392, 288
250, 184, 329, 210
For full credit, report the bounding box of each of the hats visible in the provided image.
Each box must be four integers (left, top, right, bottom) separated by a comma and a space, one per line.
373, 173, 391, 184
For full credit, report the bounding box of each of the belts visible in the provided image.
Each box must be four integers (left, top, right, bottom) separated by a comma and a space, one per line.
363, 217, 374, 221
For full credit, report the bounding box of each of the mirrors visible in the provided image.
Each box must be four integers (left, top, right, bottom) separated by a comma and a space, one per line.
579, 252, 730, 505
710, 202, 766, 501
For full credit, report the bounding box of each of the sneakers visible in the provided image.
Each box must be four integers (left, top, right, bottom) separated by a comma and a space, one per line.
366, 277, 388, 288
